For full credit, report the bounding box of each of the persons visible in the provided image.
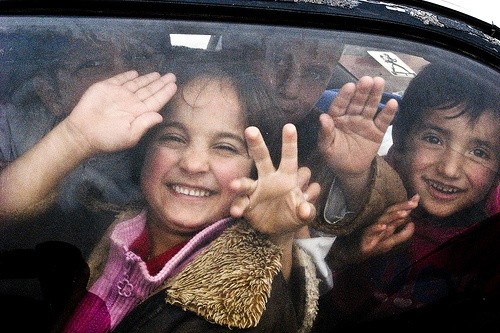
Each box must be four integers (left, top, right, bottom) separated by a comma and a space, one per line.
0, 48, 299, 333
326, 64, 500, 316
220, 24, 412, 236
0, 25, 316, 285
